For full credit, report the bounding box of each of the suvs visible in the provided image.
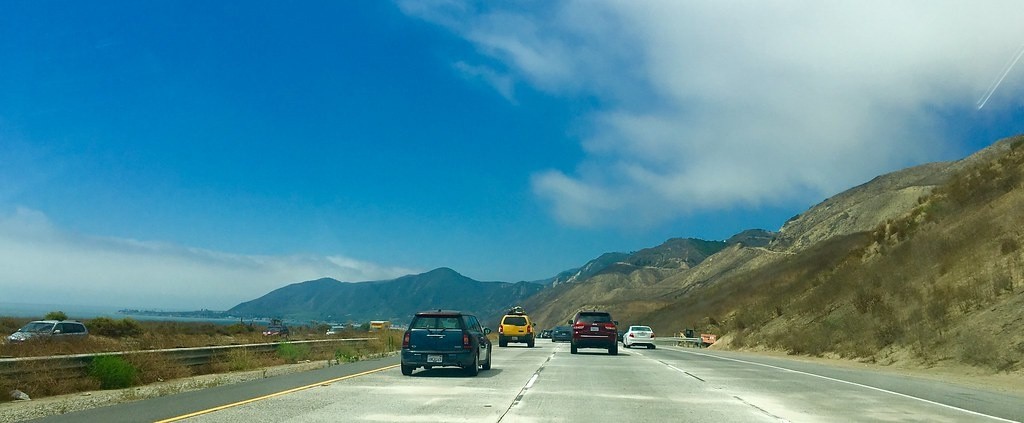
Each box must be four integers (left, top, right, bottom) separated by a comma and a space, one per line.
401, 308, 493, 378
262, 325, 290, 339
567, 310, 620, 355
498, 314, 537, 348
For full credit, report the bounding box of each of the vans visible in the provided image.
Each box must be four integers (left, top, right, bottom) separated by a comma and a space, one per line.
7, 319, 89, 347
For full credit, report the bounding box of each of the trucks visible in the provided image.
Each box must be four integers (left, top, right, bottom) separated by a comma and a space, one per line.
369, 321, 392, 334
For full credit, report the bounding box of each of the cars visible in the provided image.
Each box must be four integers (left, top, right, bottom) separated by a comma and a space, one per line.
616, 329, 626, 342
536, 328, 553, 339
326, 326, 344, 337
622, 325, 656, 350
547, 325, 573, 343
